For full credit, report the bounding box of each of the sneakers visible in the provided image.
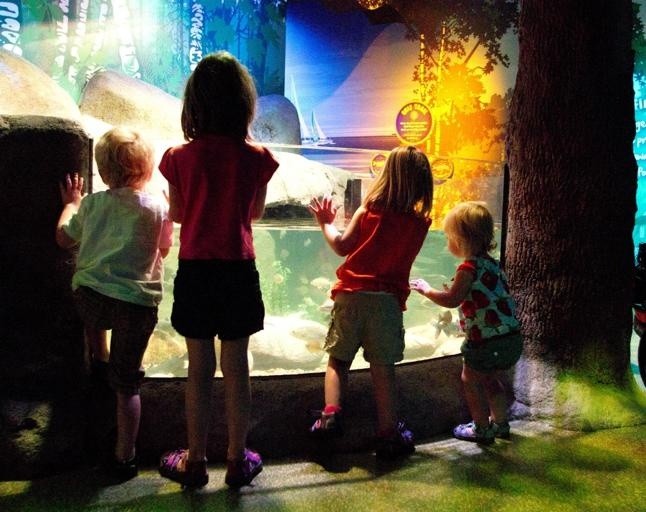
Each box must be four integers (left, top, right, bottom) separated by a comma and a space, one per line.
453, 421, 495, 444
159, 448, 209, 487
307, 411, 351, 437
224, 447, 263, 486
86, 358, 145, 385
489, 416, 510, 438
374, 431, 416, 464
110, 455, 138, 480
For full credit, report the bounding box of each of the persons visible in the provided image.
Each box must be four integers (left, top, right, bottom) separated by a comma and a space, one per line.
157, 48, 264, 490
410, 202, 522, 445
52, 127, 175, 476
306, 142, 435, 458
